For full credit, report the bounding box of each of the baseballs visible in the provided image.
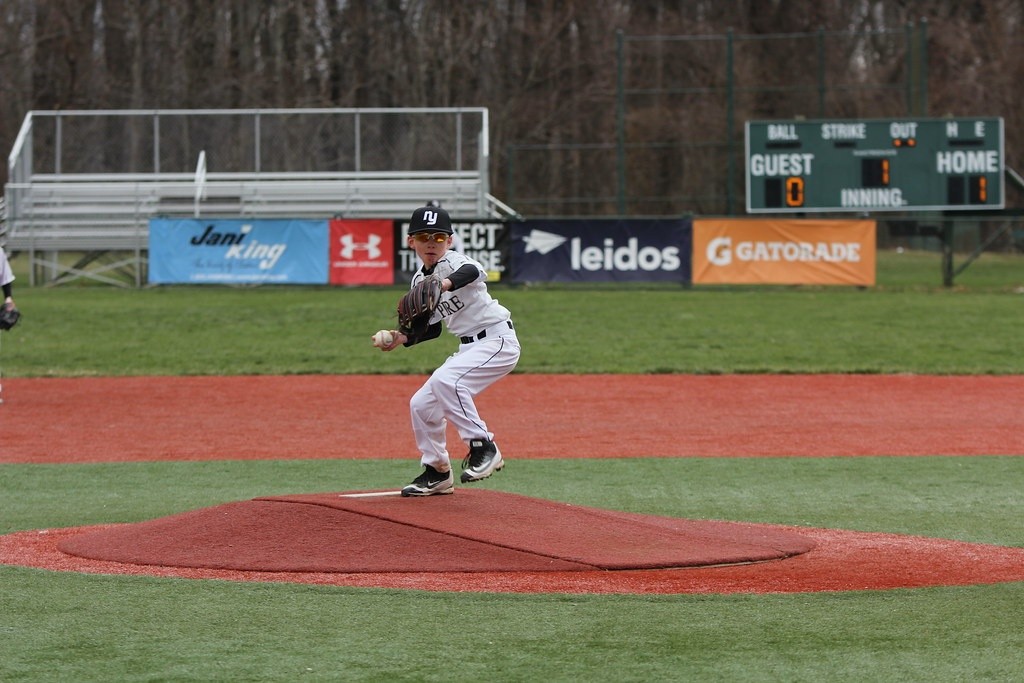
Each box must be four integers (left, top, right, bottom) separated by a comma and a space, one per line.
374, 329, 394, 349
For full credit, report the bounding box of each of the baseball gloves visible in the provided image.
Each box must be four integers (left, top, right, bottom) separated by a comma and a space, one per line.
0, 300, 22, 332
396, 273, 446, 338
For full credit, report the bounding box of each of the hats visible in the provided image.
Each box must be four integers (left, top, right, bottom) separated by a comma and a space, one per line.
408, 207, 454, 234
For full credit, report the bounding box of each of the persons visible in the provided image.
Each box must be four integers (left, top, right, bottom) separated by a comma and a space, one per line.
370, 207, 521, 496
0, 247, 15, 311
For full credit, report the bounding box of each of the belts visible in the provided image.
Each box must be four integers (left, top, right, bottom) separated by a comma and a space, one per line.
461, 321, 512, 345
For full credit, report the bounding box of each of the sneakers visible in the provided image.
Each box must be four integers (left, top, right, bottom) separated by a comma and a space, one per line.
461, 439, 505, 483
402, 464, 454, 497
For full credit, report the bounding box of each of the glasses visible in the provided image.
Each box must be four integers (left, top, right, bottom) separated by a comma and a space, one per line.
413, 232, 451, 243
